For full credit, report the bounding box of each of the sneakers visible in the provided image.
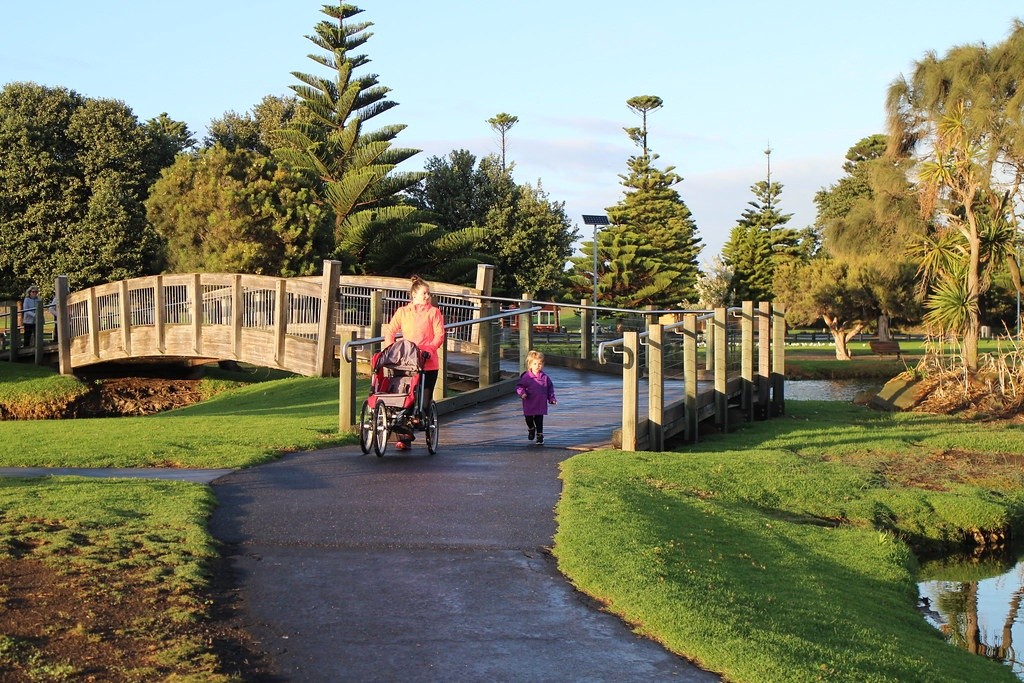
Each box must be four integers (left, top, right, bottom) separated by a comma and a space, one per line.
536, 434, 544, 445
528, 427, 535, 440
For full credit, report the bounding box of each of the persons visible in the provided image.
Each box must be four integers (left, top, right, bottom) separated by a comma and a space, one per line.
22, 285, 71, 349
515, 350, 557, 445
385, 274, 447, 451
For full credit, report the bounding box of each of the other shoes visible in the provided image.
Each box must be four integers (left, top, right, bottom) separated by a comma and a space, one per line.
395, 440, 412, 450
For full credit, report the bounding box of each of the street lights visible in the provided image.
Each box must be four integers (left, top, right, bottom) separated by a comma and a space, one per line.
582, 214, 610, 348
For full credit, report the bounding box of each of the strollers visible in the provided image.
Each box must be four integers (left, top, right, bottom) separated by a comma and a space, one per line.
360, 339, 439, 457
